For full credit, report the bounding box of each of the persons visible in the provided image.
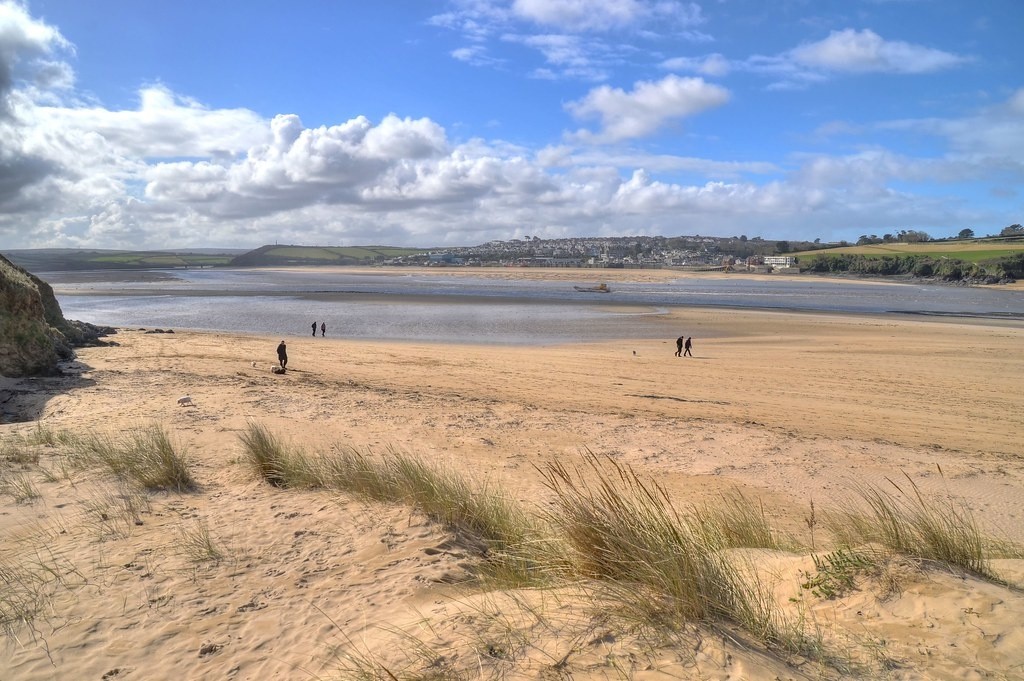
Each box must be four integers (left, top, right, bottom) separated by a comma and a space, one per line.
311, 322, 317, 337
321, 322, 326, 336
675, 335, 683, 357
277, 341, 288, 370
684, 337, 692, 356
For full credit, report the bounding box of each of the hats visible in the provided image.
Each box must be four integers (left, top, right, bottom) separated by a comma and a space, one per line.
681, 336, 684, 338
689, 337, 691, 339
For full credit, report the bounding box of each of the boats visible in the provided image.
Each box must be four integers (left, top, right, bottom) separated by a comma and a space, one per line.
573, 284, 611, 293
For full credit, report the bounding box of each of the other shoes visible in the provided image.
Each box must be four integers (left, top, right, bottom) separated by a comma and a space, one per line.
684, 354, 686, 356
285, 368, 287, 370
675, 352, 682, 357
690, 355, 692, 356
322, 334, 325, 336
312, 334, 315, 336
